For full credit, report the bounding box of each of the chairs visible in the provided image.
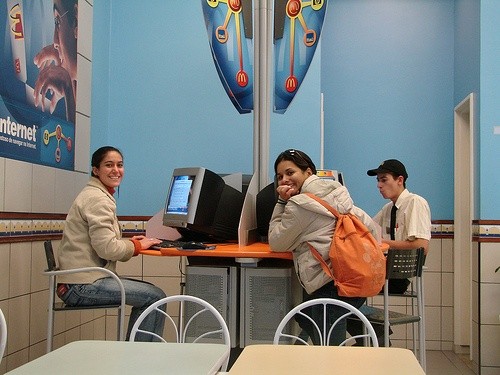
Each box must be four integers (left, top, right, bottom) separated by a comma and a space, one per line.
129, 295, 229, 373
351, 247, 427, 372
42, 241, 127, 353
273, 297, 379, 348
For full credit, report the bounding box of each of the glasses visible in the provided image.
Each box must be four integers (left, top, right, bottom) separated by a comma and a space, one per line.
284, 149, 308, 165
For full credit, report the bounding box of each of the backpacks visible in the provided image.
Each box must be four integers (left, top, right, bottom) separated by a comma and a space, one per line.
299, 189, 386, 298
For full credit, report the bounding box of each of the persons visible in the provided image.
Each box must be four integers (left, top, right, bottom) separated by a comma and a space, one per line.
56, 146, 167, 342
34, 0, 78, 123
346, 159, 432, 347
269, 149, 380, 346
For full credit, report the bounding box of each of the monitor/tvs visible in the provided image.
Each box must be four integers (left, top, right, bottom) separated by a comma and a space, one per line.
161, 166, 345, 243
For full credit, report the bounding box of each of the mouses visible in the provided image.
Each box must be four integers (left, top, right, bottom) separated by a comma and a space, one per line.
182, 241, 206, 249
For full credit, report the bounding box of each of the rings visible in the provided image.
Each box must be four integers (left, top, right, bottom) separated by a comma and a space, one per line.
148, 238, 153, 241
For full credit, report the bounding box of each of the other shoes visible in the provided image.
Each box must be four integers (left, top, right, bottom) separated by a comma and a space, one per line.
352, 335, 392, 347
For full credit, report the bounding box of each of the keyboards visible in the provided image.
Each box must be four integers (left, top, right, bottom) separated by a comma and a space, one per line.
152, 239, 185, 251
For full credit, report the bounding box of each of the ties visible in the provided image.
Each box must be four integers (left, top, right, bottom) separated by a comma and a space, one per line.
390, 205, 398, 240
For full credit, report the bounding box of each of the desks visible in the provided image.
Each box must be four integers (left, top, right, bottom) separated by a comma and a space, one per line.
228, 344, 427, 375
138, 243, 390, 348
3, 340, 227, 375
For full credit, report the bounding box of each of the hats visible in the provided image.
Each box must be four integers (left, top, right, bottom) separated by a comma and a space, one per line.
366, 159, 408, 179
